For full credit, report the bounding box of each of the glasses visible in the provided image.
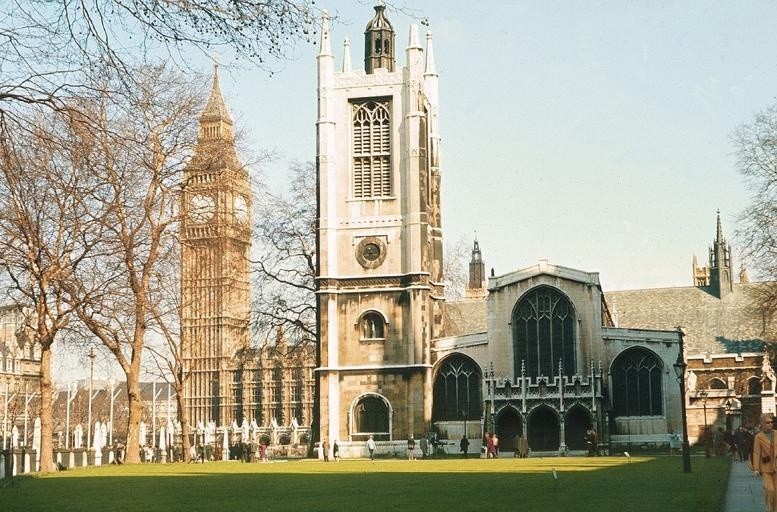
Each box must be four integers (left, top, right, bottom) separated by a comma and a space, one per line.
764, 421, 774, 424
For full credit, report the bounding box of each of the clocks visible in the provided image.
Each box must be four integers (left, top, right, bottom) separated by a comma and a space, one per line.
187, 193, 217, 226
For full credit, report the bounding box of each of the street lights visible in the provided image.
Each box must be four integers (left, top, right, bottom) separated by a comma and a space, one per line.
700, 389, 711, 458
460, 404, 470, 458
86, 346, 96, 450
671, 354, 692, 472
725, 399, 731, 434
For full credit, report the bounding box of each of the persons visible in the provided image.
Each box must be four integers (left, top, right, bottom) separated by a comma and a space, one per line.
592, 428, 599, 457
750, 412, 776, 512
583, 429, 593, 456
513, 433, 528, 458
482, 432, 499, 459
259, 441, 268, 462
366, 435, 377, 462
111, 440, 222, 464
321, 437, 339, 461
232, 437, 259, 463
407, 432, 440, 461
459, 434, 471, 458
668, 422, 776, 468
